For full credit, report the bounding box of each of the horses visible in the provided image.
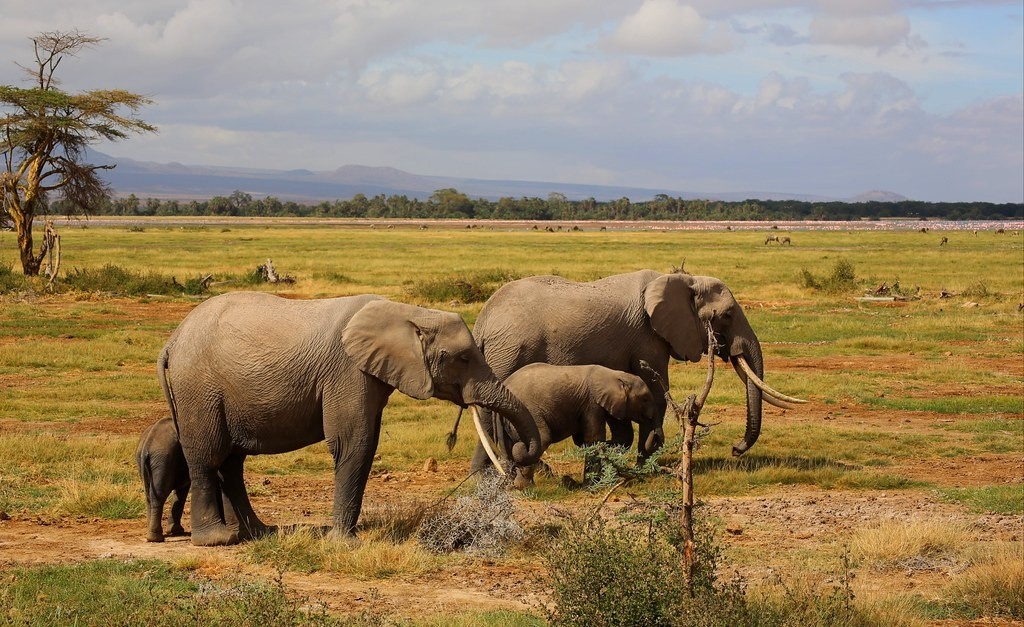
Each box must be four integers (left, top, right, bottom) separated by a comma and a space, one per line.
370, 223, 1019, 248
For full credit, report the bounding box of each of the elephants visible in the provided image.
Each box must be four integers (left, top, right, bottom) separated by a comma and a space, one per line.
135, 415, 190, 543
157, 290, 542, 547
445, 269, 809, 492
